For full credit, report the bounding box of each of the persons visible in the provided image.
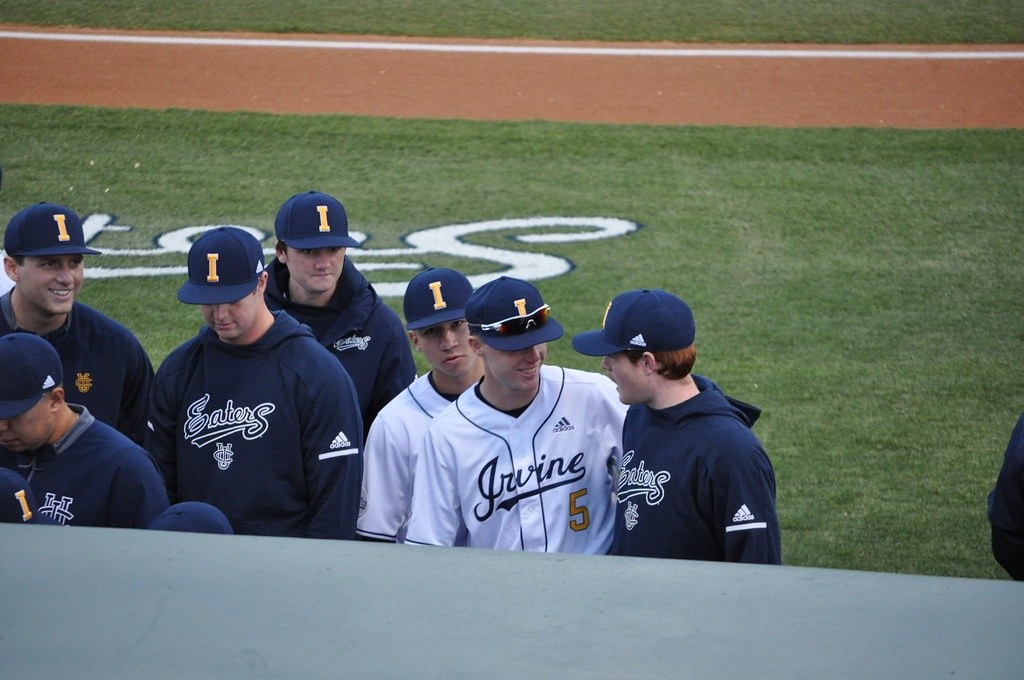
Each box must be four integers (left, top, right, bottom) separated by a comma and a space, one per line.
2, 202, 156, 450
356, 267, 486, 542
403, 276, 630, 556
988, 413, 1024, 581
151, 500, 233, 535
573, 290, 781, 565
263, 190, 416, 450
144, 226, 364, 540
0, 332, 172, 530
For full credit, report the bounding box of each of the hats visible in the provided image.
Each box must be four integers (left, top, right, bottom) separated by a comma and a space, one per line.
0, 333, 64, 420
572, 289, 695, 357
148, 501, 234, 536
4, 201, 102, 257
1, 467, 67, 526
275, 191, 360, 250
403, 268, 474, 330
176, 227, 266, 304
464, 276, 564, 353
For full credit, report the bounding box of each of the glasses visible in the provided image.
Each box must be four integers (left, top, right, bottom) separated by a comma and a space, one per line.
466, 303, 550, 337
